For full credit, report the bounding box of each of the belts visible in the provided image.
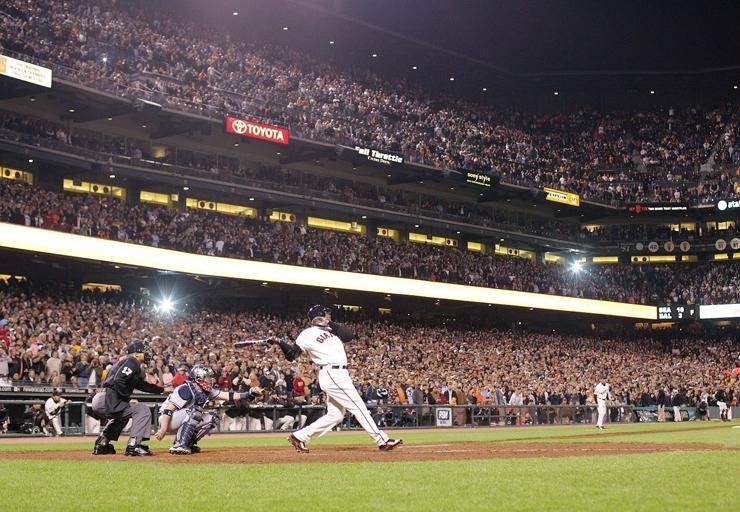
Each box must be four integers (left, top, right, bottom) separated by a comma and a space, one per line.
320, 365, 346, 369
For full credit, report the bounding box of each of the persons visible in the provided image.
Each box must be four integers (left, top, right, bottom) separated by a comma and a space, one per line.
154, 361, 267, 456
0, 2, 739, 436
267, 303, 405, 454
87, 339, 176, 457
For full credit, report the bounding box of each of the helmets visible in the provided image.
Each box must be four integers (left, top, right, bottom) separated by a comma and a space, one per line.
127, 340, 151, 364
308, 305, 331, 320
189, 364, 214, 393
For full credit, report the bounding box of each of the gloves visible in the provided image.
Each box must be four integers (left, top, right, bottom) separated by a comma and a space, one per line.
267, 336, 281, 345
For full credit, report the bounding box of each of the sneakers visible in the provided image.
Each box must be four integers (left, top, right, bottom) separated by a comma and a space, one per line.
169, 443, 192, 455
94, 444, 116, 454
379, 438, 402, 451
286, 434, 309, 452
125, 444, 152, 456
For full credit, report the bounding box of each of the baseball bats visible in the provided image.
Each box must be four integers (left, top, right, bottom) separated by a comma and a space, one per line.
235, 341, 267, 349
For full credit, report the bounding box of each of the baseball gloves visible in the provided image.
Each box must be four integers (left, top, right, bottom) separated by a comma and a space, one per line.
249, 387, 263, 402
